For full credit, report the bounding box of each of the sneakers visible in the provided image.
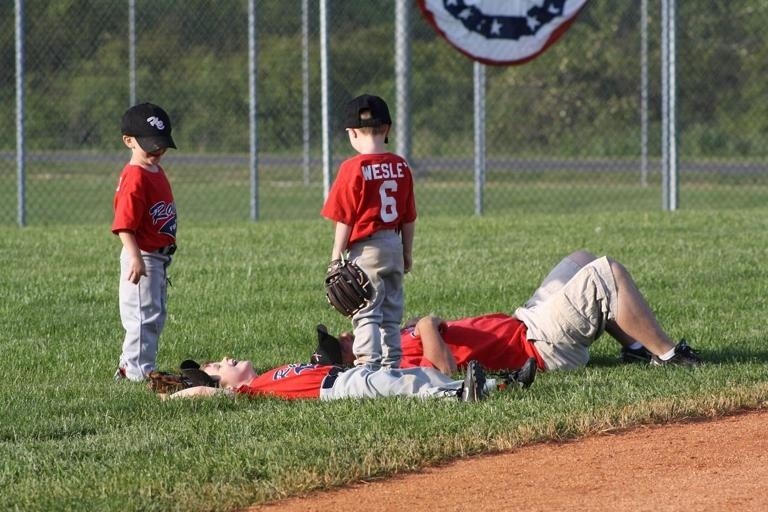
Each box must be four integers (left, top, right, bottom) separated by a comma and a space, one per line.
650, 338, 710, 371
462, 360, 485, 404
624, 346, 657, 364
506, 359, 537, 391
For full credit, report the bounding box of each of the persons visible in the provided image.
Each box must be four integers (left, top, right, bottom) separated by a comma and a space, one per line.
111, 101, 178, 383
311, 249, 702, 373
321, 95, 418, 368
159, 355, 537, 404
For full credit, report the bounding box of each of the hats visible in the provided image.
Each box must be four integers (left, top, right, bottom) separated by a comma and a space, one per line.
343, 94, 392, 130
180, 359, 212, 388
309, 324, 343, 368
121, 103, 177, 153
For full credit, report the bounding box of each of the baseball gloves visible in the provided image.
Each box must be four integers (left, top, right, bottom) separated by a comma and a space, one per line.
325, 258, 376, 317
149, 370, 193, 393
